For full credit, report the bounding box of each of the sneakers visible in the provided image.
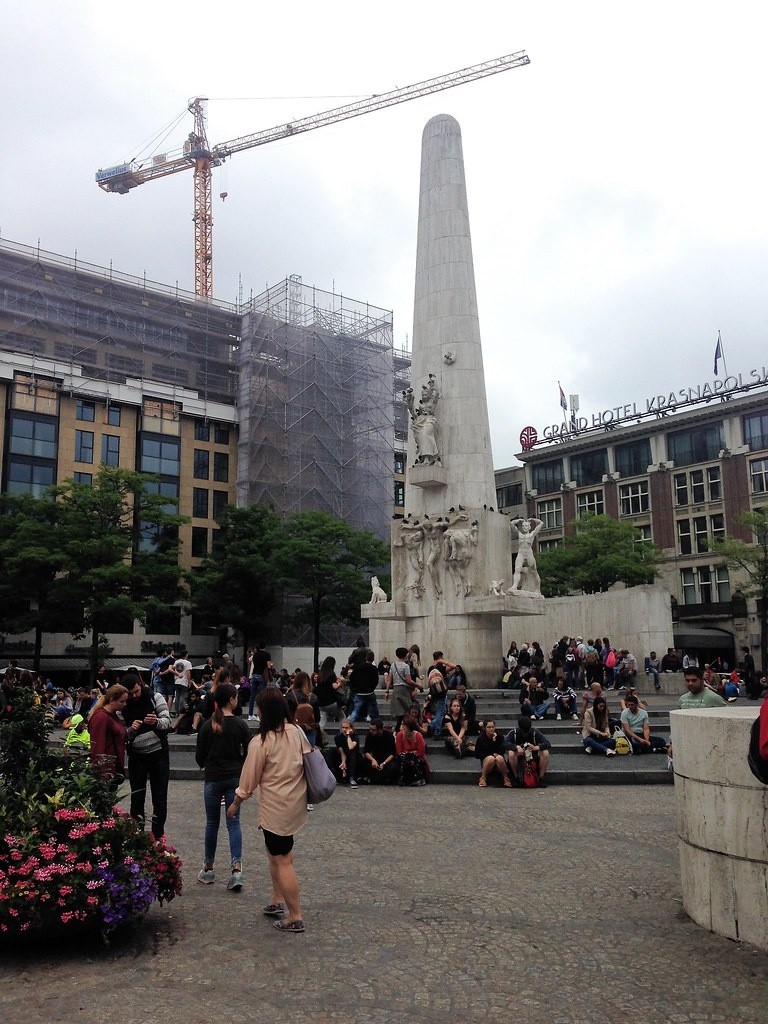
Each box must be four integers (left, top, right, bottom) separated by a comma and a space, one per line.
226, 872, 242, 890
197, 869, 216, 884
557, 714, 561, 720
571, 713, 578, 721
349, 780, 358, 789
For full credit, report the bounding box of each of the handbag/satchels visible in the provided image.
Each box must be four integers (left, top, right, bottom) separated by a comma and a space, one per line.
613, 725, 633, 754
428, 669, 448, 694
293, 722, 336, 804
62, 717, 71, 728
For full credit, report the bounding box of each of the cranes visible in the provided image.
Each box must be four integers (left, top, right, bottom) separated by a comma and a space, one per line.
93, 48, 534, 304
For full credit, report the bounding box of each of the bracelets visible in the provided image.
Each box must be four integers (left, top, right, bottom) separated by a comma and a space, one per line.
346, 734, 349, 738
233, 795, 241, 806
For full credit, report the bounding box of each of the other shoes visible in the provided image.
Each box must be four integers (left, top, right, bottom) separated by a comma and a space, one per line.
530, 714, 536, 720
479, 778, 486, 787
455, 746, 462, 759
366, 714, 372, 722
606, 748, 616, 757
189, 729, 198, 735
247, 714, 261, 721
503, 778, 512, 787
538, 776, 547, 788
539, 716, 544, 720
262, 904, 284, 913
273, 920, 305, 931
585, 746, 592, 753
306, 802, 314, 811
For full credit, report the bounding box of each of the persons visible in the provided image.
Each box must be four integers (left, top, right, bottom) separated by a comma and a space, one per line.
0, 660, 87, 729
404, 380, 440, 467
475, 718, 512, 788
444, 700, 475, 758
294, 704, 431, 789
505, 717, 551, 788
408, 644, 484, 741
386, 648, 423, 735
644, 646, 768, 702
120, 667, 170, 842
241, 634, 391, 729
668, 666, 730, 759
195, 683, 252, 891
519, 677, 647, 727
63, 714, 91, 753
582, 697, 616, 756
398, 515, 479, 598
87, 644, 241, 734
501, 636, 638, 691
87, 684, 129, 812
620, 696, 667, 755
509, 518, 544, 597
226, 687, 311, 933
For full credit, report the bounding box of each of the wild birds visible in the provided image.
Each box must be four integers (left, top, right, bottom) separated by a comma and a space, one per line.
402, 373, 448, 469
392, 503, 537, 526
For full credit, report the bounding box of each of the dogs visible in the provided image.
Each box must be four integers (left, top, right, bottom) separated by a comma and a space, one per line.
368, 576, 388, 605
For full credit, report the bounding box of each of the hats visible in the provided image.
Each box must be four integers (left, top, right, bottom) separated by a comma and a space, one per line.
69, 714, 83, 729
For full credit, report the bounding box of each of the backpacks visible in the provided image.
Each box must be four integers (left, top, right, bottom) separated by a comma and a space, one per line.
585, 648, 598, 664
604, 651, 615, 668
400, 751, 426, 786
565, 649, 576, 665
